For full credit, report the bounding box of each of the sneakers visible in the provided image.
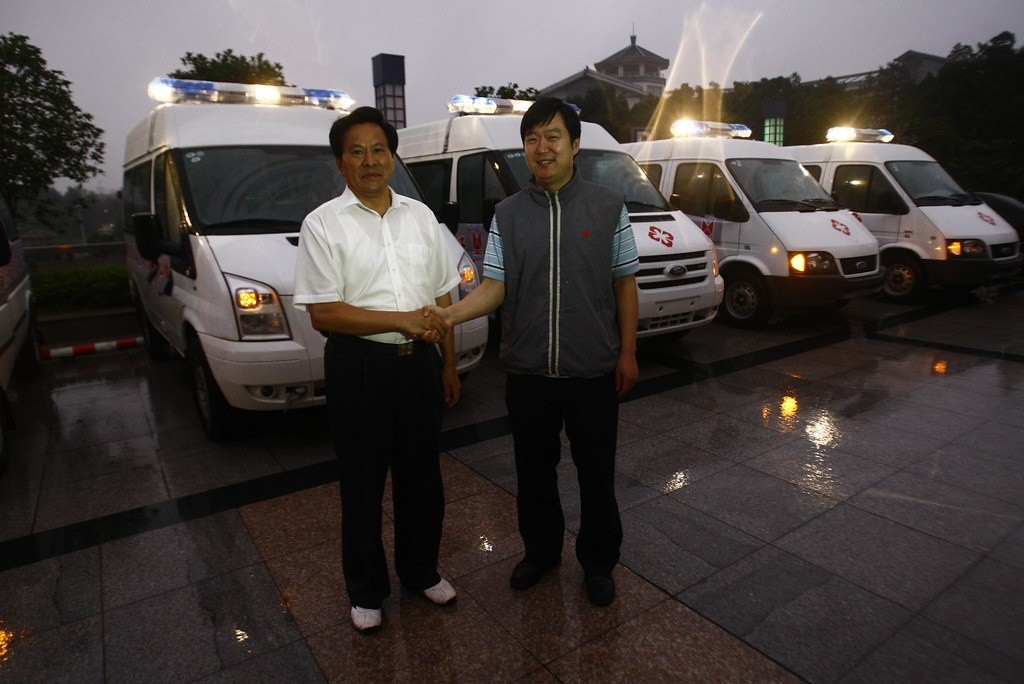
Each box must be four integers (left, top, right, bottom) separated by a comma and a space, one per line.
423, 576, 458, 608
585, 571, 616, 607
510, 553, 564, 591
350, 605, 382, 633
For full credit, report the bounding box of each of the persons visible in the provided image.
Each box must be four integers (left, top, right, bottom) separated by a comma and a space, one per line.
405, 95, 641, 606
294, 106, 463, 633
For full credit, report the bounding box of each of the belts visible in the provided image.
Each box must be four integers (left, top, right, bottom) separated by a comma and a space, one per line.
327, 332, 435, 357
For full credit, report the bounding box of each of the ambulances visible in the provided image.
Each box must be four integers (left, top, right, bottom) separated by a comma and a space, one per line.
619, 119, 887, 327
121, 77, 487, 438
778, 125, 1023, 303
395, 93, 725, 341
